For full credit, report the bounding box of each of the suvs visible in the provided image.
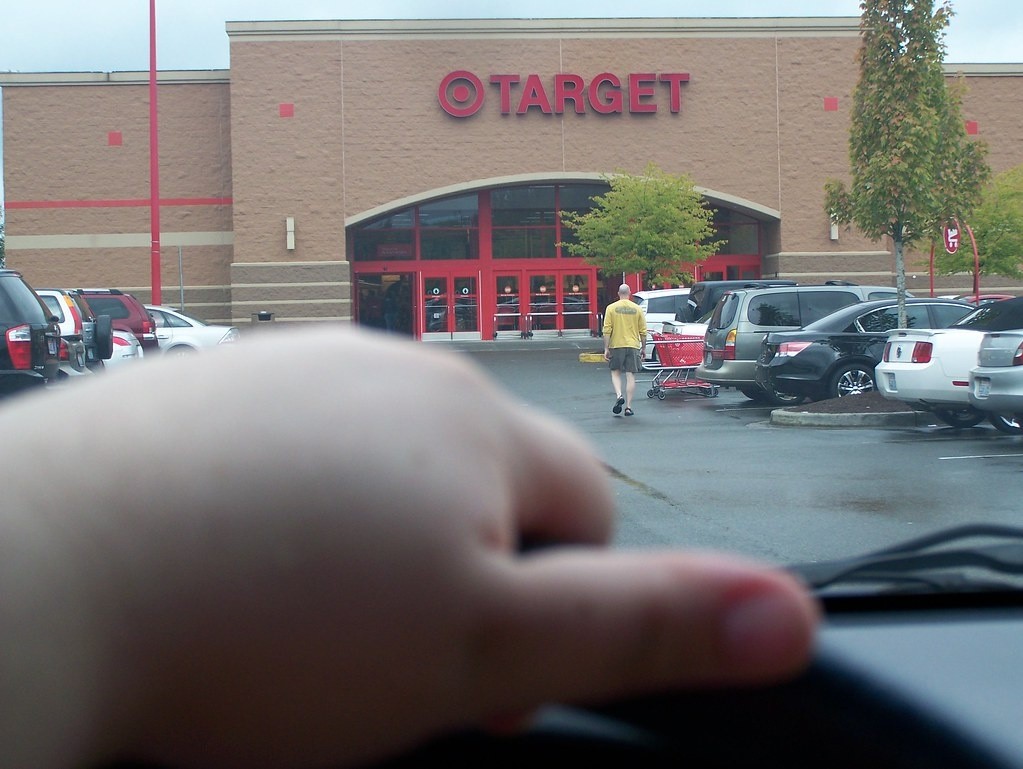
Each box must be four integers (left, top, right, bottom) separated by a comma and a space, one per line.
0, 269, 62, 398
695, 280, 924, 405
673, 279, 802, 327
31, 287, 167, 390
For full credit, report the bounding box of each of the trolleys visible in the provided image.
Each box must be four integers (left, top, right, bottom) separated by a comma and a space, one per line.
642, 327, 721, 400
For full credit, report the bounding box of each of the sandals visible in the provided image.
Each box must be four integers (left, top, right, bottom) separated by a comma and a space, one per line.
625, 407, 634, 416
613, 395, 625, 414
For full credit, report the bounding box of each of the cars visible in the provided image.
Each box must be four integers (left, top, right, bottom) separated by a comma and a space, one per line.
632, 285, 691, 362
141, 304, 240, 366
752, 295, 982, 401
952, 294, 1016, 311
501, 296, 590, 311
875, 296, 1023, 434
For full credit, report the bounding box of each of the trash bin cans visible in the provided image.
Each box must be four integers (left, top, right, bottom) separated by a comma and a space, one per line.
251, 311, 275, 326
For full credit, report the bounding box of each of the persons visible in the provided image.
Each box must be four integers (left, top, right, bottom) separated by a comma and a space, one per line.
0, 322, 822, 769
602, 284, 647, 416
